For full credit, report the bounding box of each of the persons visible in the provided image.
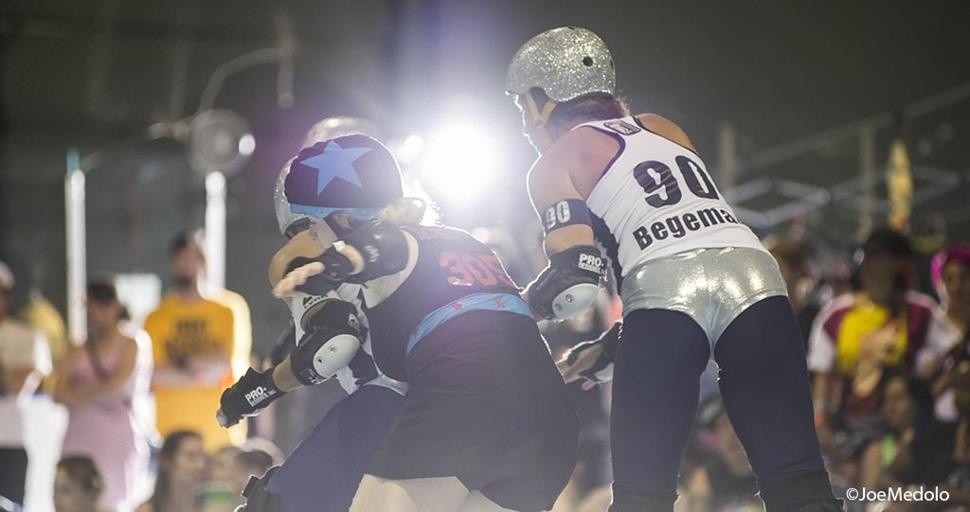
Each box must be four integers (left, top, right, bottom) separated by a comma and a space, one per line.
0, 229, 970, 512
503, 25, 841, 512
216, 132, 578, 512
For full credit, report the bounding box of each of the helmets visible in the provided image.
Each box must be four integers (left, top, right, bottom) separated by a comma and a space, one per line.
503, 27, 616, 104
274, 117, 402, 236
